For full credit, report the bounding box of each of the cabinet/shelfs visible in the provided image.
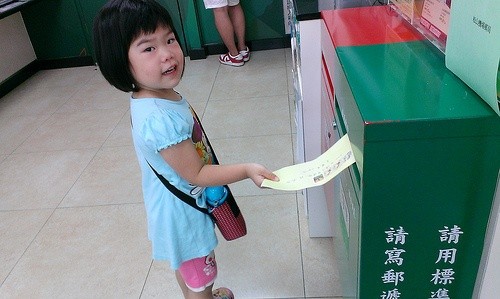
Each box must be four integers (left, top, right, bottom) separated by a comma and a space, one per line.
319, 7, 500, 299
287, 1, 336, 237
21, 0, 187, 61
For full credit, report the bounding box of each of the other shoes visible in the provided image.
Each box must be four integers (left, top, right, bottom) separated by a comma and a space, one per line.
212, 287, 234, 299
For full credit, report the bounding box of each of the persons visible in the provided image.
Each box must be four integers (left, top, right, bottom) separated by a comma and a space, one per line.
93, 0, 280, 299
202, 0, 250, 66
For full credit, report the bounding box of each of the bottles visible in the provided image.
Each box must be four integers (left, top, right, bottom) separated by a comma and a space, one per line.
204, 184, 228, 213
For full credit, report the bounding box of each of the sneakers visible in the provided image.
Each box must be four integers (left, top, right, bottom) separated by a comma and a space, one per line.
219, 53, 244, 66
239, 47, 250, 61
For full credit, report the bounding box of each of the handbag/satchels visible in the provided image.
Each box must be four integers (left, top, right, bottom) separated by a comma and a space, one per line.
210, 185, 246, 241
